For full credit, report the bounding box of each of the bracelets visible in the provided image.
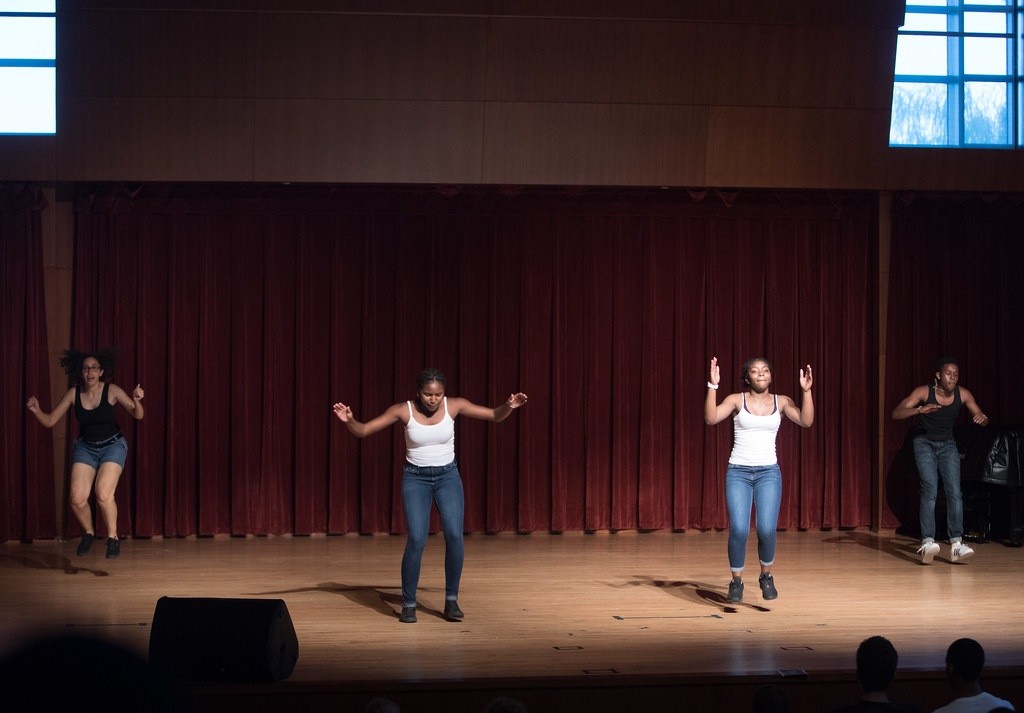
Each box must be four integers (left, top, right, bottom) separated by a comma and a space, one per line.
707, 381, 719, 389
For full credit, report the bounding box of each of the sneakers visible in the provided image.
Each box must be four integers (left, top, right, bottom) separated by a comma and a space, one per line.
917, 542, 940, 564
727, 577, 744, 602
105, 537, 120, 558
77, 534, 96, 554
759, 574, 777, 600
950, 542, 974, 562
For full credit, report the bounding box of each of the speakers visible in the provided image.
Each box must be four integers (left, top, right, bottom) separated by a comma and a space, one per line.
147, 596, 298, 683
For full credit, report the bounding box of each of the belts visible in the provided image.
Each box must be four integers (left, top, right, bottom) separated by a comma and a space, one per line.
87, 433, 122, 447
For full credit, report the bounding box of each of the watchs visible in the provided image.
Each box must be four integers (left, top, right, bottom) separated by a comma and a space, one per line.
918, 406, 922, 414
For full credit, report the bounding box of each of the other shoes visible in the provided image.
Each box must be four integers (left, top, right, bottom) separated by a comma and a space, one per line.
444, 600, 464, 619
400, 607, 417, 622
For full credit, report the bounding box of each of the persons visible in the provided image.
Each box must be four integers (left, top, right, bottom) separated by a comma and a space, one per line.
366, 698, 401, 713
333, 368, 528, 622
487, 696, 528, 713
892, 364, 989, 563
26, 352, 145, 558
752, 636, 1015, 713
701, 356, 815, 600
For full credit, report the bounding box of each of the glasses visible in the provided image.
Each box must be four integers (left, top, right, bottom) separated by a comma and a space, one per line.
81, 366, 101, 372
